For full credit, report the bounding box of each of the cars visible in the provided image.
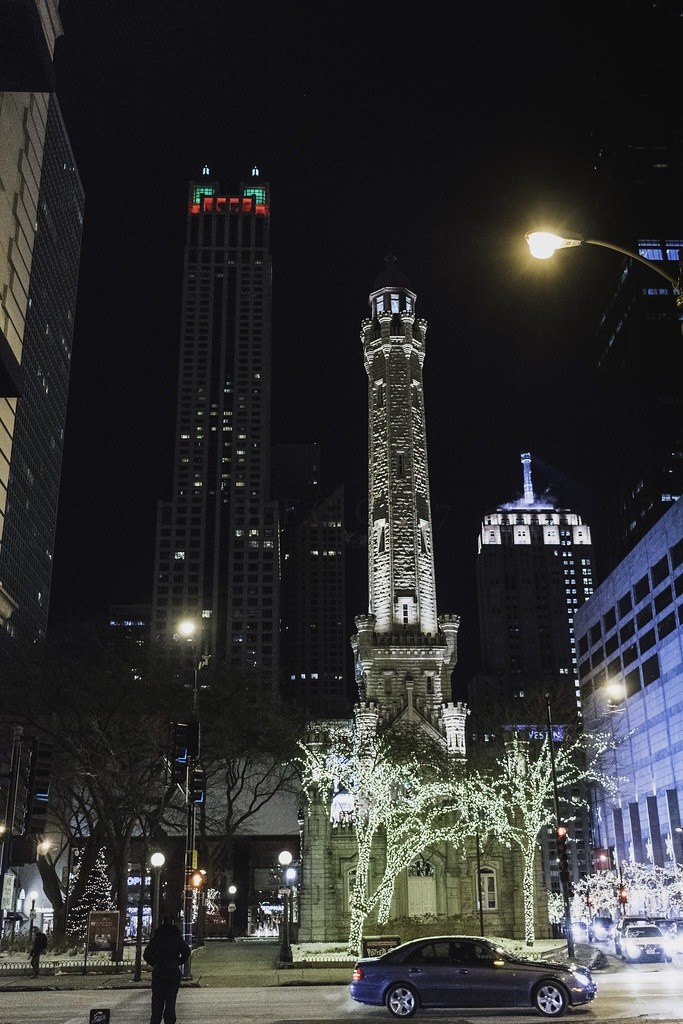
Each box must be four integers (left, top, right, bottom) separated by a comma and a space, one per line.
349, 936, 598, 1019
559, 915, 683, 964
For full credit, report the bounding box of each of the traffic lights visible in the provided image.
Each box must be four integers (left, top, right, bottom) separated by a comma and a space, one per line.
186, 765, 205, 804
26, 737, 54, 835
173, 722, 197, 783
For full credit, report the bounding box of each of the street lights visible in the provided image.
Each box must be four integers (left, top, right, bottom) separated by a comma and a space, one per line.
278, 850, 293, 962
228, 885, 237, 942
29, 891, 38, 945
151, 852, 165, 940
176, 619, 203, 988
286, 868, 296, 944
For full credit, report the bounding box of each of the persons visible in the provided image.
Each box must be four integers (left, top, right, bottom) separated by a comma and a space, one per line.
27, 927, 44, 979
143, 914, 190, 1024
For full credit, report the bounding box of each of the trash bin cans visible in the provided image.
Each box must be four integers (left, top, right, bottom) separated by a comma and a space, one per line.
278, 922, 298, 943
362, 934, 401, 958
